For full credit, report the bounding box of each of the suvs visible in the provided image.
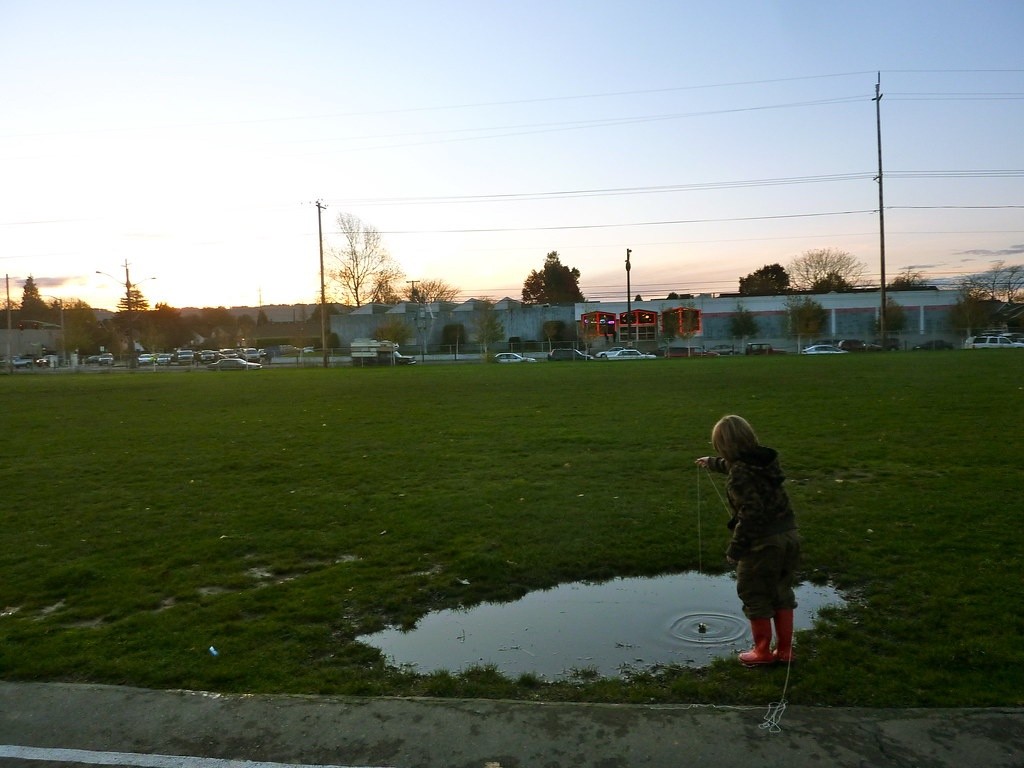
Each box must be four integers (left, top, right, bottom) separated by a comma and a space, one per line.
745, 343, 787, 354
547, 349, 593, 361
965, 336, 1024, 349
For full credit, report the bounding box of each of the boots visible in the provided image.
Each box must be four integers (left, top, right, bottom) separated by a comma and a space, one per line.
738, 608, 795, 664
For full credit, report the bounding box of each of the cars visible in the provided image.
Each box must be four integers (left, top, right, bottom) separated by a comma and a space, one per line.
912, 340, 955, 351
838, 339, 882, 352
136, 345, 273, 366
4, 350, 116, 370
802, 345, 847, 353
207, 358, 261, 370
494, 353, 536, 362
708, 344, 740, 355
595, 347, 627, 359
873, 337, 904, 352
664, 347, 721, 358
645, 346, 688, 356
803, 339, 842, 350
606, 349, 656, 360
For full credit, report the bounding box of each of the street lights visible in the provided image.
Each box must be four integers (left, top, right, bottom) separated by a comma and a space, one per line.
95, 270, 156, 369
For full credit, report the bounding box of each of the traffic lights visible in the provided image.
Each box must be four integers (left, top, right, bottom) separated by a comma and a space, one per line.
19, 324, 23, 331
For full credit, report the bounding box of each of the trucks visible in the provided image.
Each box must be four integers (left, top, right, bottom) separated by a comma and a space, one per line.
350, 339, 417, 366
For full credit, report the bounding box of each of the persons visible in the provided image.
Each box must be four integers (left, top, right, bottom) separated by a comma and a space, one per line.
695, 415, 799, 665
